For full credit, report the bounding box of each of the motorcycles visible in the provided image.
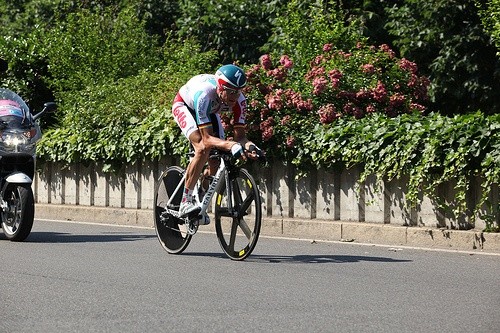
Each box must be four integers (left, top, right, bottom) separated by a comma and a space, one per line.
0, 88, 59, 243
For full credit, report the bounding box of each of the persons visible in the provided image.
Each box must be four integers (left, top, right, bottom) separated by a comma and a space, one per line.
171, 65, 264, 226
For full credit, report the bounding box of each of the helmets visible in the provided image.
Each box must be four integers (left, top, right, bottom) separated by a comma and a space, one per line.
215, 64, 247, 91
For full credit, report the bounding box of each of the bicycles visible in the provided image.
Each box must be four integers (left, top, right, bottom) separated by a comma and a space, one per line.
153, 144, 262, 261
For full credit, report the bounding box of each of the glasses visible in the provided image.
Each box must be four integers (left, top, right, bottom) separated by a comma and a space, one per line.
226, 89, 241, 95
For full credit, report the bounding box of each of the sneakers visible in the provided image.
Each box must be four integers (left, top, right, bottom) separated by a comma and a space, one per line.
204, 211, 210, 224
179, 202, 201, 218
